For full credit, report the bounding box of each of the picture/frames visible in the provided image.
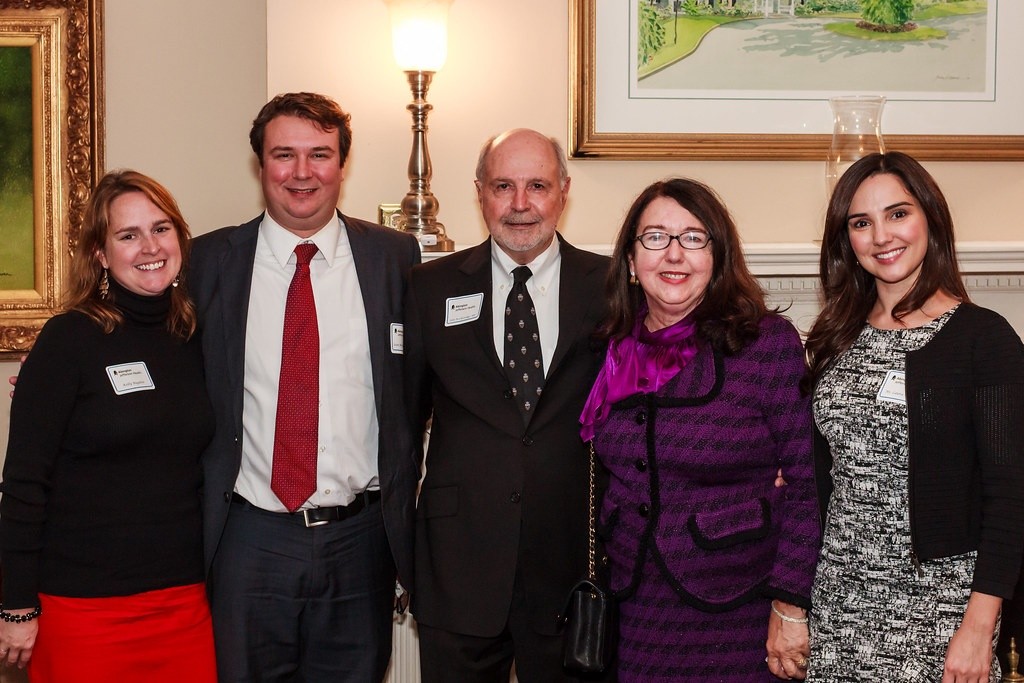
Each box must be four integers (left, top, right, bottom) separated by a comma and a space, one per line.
0, 0, 106, 361
567, 0, 1024, 162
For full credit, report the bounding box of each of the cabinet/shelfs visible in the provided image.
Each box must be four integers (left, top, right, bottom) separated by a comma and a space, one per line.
384, 242, 1024, 683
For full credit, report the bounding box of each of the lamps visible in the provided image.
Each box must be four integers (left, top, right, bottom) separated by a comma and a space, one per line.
387, 1, 456, 253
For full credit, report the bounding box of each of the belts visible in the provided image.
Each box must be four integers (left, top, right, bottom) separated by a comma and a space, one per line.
231, 490, 385, 528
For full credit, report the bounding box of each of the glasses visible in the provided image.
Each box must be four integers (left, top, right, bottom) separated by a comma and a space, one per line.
631, 230, 713, 251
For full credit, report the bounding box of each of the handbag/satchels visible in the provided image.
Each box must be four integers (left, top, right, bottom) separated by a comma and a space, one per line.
571, 557, 607, 673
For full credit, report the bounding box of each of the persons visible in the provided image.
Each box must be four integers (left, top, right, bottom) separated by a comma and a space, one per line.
774, 150, 1024, 682
579, 176, 823, 683
174, 89, 423, 681
406, 128, 615, 683
0, 168, 216, 681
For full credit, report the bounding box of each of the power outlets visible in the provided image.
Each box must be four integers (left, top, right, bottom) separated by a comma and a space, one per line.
378, 204, 408, 232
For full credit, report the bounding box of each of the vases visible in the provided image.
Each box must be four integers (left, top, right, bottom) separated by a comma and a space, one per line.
812, 95, 887, 247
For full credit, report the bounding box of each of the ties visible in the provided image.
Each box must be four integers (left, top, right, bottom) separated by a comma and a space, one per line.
271, 245, 319, 513
503, 267, 545, 432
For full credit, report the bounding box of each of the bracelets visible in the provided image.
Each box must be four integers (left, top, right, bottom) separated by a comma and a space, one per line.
0, 605, 44, 623
771, 601, 808, 623
